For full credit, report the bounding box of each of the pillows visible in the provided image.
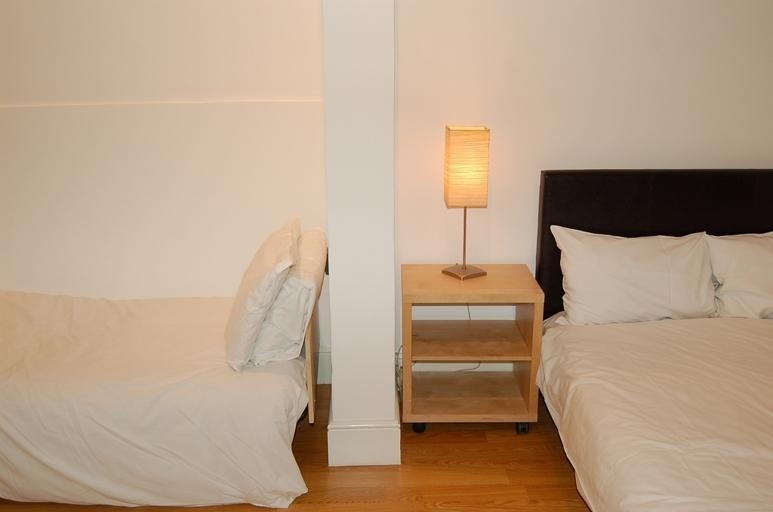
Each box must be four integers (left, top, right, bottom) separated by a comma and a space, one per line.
218, 217, 328, 373
545, 219, 771, 327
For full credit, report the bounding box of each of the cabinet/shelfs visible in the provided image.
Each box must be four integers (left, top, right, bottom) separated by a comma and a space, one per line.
400, 263, 545, 435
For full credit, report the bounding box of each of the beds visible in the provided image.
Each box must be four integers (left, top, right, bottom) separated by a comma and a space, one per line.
533, 168, 772, 512
0, 290, 316, 508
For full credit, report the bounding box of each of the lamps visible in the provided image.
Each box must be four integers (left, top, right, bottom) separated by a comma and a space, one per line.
440, 124, 490, 280
